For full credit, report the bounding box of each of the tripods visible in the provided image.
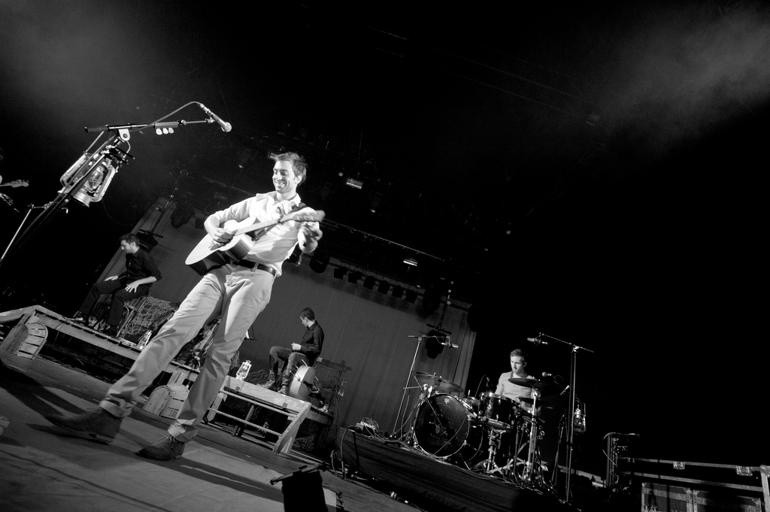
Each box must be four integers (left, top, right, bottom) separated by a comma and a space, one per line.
390, 400, 546, 488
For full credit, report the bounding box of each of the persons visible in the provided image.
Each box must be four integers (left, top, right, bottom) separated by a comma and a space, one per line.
71, 233, 162, 337
261, 308, 324, 395
44, 152, 323, 460
495, 349, 541, 417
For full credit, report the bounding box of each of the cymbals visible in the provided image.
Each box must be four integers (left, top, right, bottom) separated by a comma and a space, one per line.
414, 371, 463, 394
509, 377, 539, 387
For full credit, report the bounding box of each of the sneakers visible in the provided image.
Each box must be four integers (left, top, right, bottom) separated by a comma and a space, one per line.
278, 385, 289, 395
257, 381, 277, 390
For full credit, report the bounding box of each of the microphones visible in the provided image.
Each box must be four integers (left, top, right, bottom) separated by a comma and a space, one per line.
200, 103, 231, 135
527, 337, 549, 345
442, 341, 459, 349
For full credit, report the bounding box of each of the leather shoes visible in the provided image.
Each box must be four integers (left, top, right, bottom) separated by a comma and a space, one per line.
100, 326, 117, 337
68, 315, 87, 325
136, 433, 185, 462
39, 406, 122, 444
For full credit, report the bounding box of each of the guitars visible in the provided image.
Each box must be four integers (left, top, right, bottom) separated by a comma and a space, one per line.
185, 210, 325, 276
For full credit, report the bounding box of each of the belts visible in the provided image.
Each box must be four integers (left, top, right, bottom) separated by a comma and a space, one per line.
233, 260, 276, 276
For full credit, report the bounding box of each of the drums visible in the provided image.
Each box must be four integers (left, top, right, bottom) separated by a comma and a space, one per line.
414, 393, 487, 463
480, 391, 518, 430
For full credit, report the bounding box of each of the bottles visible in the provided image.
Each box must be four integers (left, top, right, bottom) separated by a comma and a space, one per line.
235, 358, 252, 381
136, 328, 153, 349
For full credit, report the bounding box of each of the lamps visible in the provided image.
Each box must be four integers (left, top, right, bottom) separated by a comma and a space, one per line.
333, 264, 419, 308
56, 129, 135, 211
403, 258, 419, 266
345, 176, 362, 188
170, 201, 302, 271
308, 247, 331, 274
